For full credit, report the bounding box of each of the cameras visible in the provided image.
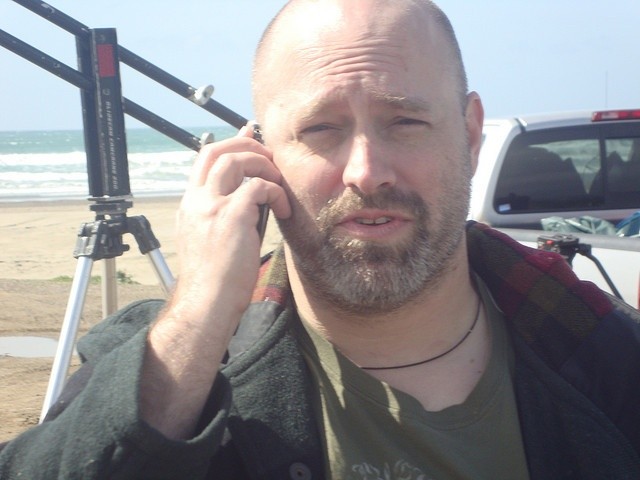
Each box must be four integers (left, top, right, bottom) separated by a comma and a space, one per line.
536, 233, 593, 271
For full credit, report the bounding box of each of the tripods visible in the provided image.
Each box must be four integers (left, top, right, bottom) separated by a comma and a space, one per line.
0, 0, 253, 425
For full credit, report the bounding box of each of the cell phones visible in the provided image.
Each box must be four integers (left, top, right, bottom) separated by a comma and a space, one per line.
252, 124, 271, 237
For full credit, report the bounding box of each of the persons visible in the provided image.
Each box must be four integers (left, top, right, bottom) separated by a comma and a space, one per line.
0, 0, 639, 479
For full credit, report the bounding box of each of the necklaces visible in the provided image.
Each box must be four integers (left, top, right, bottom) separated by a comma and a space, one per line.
356, 295, 483, 377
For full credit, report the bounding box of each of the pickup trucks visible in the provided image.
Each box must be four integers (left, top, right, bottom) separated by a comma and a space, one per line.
468, 109, 640, 309
